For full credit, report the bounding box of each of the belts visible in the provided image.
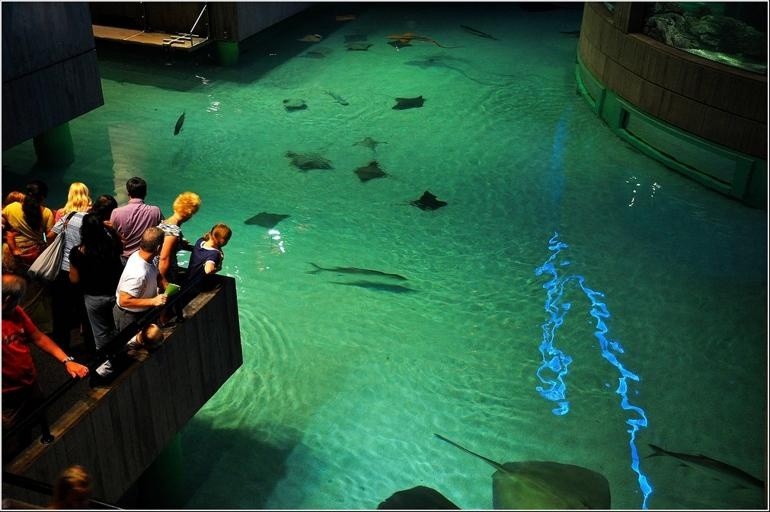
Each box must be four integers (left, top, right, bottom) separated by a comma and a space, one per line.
118, 307, 142, 315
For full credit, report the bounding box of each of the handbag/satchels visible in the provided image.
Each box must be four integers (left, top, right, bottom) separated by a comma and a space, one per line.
27, 210, 78, 284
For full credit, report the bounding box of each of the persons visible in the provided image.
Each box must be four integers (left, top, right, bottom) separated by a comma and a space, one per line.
3, 174, 235, 464
